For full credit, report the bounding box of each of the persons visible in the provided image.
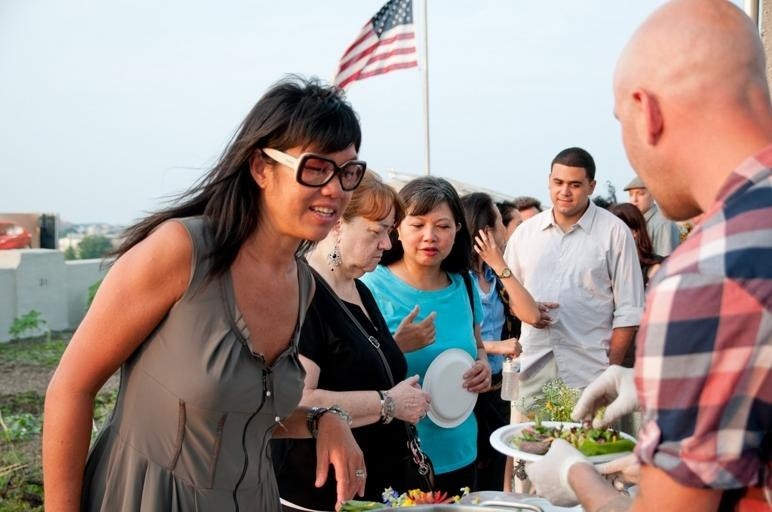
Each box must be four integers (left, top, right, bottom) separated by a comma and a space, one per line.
623, 171, 682, 288
512, 194, 543, 223
359, 174, 494, 489
606, 202, 666, 364
517, 0, 772, 512
493, 199, 524, 344
500, 145, 649, 496
461, 189, 542, 488
268, 166, 438, 512
32, 75, 371, 512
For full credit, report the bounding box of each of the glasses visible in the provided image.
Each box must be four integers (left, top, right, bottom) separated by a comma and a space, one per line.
263, 147, 368, 191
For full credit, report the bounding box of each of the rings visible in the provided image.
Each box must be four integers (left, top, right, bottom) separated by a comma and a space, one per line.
363, 473, 367, 479
355, 469, 363, 477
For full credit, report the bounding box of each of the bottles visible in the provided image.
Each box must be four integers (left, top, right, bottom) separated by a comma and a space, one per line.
501, 353, 520, 401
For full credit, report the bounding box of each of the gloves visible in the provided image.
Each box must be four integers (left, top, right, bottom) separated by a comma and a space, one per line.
571, 364, 635, 430
525, 438, 596, 508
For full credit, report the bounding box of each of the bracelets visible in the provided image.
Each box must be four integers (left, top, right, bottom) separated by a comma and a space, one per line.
305, 403, 353, 437
374, 388, 396, 425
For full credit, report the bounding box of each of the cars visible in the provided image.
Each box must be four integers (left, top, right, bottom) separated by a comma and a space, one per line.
0, 222, 31, 248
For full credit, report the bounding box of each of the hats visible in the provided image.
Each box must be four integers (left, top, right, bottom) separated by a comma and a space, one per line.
623, 176, 647, 191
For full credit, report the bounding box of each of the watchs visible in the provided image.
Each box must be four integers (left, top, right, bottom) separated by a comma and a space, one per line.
495, 267, 515, 283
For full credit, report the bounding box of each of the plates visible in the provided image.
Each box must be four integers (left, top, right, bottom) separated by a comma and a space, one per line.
422, 347, 480, 429
489, 421, 638, 464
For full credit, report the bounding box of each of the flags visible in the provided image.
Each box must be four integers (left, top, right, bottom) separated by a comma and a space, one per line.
328, 1, 421, 93
